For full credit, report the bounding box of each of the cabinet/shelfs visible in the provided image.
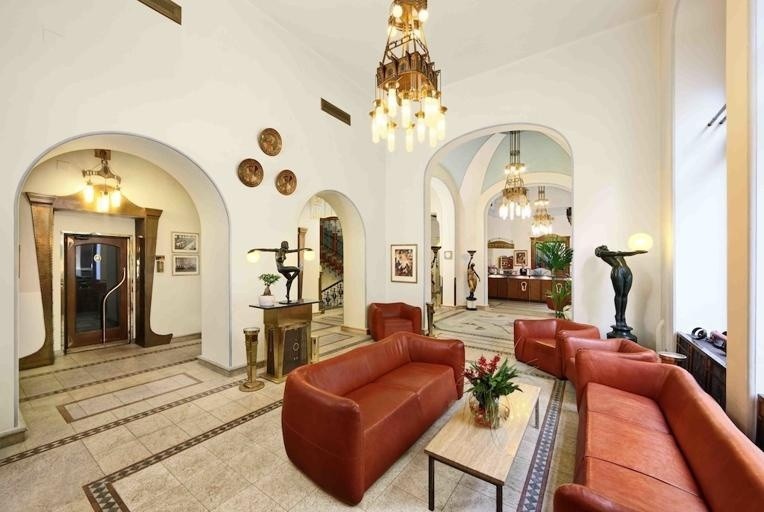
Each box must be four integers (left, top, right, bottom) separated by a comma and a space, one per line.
677, 332, 727, 415
490, 274, 571, 304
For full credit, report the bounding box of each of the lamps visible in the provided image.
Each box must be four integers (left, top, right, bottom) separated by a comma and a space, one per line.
497, 129, 536, 221
77, 148, 125, 214
528, 185, 555, 238
366, 0, 455, 156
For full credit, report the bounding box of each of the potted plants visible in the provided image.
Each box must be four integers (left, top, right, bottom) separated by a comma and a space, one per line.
257, 273, 279, 305
532, 239, 573, 310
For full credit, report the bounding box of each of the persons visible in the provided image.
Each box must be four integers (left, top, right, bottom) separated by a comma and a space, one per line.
431, 258, 436, 300
247, 242, 313, 303
520, 264, 527, 275
594, 245, 648, 327
467, 256, 480, 298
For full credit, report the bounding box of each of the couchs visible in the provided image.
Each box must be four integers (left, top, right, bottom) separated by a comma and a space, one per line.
553, 351, 764, 511
282, 329, 468, 508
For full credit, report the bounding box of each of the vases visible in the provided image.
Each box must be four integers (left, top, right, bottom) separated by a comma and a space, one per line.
470, 396, 499, 426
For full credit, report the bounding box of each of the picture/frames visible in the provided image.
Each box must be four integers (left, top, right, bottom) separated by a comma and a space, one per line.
513, 250, 528, 266
171, 251, 201, 277
170, 231, 200, 253
389, 243, 419, 284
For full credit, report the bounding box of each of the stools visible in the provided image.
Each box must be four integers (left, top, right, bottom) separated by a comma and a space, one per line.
658, 348, 686, 365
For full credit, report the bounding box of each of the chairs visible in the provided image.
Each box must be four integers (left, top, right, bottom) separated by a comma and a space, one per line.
568, 335, 658, 388
367, 301, 424, 341
510, 318, 600, 379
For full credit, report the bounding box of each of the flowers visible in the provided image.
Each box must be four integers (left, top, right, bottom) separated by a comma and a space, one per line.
447, 332, 540, 449
